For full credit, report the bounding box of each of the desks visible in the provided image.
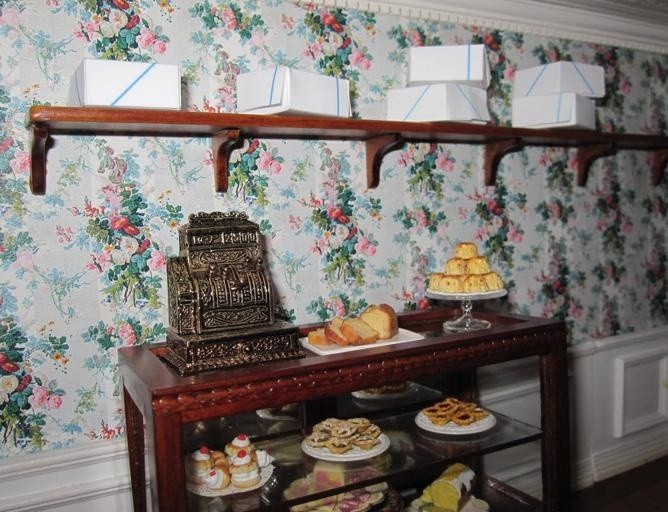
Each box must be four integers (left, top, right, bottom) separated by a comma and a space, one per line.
118, 307, 567, 512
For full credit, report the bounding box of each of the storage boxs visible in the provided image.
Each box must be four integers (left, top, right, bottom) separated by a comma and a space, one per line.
513, 62, 605, 97
234, 67, 353, 119
69, 58, 181, 110
386, 83, 490, 124
512, 93, 597, 133
408, 44, 491, 90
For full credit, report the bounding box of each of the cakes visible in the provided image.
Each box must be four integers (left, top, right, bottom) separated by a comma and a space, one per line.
186, 431, 277, 490
283, 461, 390, 512
418, 462, 477, 512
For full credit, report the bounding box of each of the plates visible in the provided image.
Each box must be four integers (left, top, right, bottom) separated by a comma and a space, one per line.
353, 380, 419, 401
302, 432, 391, 462
189, 463, 274, 498
283, 469, 389, 511
256, 406, 298, 422
298, 327, 425, 358
416, 410, 496, 435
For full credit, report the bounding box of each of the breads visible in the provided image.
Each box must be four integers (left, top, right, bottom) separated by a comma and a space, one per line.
307, 305, 400, 347
426, 242, 505, 293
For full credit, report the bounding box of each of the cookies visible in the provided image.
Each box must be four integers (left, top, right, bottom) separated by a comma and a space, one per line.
423, 396, 488, 426
305, 415, 383, 453
